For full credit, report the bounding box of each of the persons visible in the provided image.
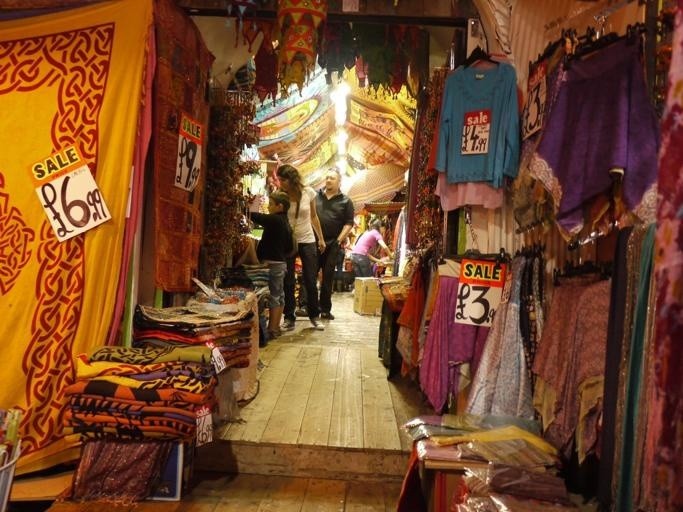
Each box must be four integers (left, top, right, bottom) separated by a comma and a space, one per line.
294, 166, 353, 320
241, 191, 293, 337
275, 164, 328, 331
350, 220, 393, 296
330, 235, 349, 293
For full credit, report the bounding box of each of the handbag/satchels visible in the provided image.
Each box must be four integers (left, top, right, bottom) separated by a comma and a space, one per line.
285, 227, 299, 259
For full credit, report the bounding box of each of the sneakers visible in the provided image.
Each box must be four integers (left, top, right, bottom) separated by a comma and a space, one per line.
321, 310, 335, 320
309, 316, 325, 330
278, 317, 296, 331
295, 306, 309, 317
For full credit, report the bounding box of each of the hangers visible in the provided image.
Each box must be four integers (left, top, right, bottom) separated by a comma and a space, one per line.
527, 15, 641, 73
461, 30, 499, 70
432, 236, 615, 286
